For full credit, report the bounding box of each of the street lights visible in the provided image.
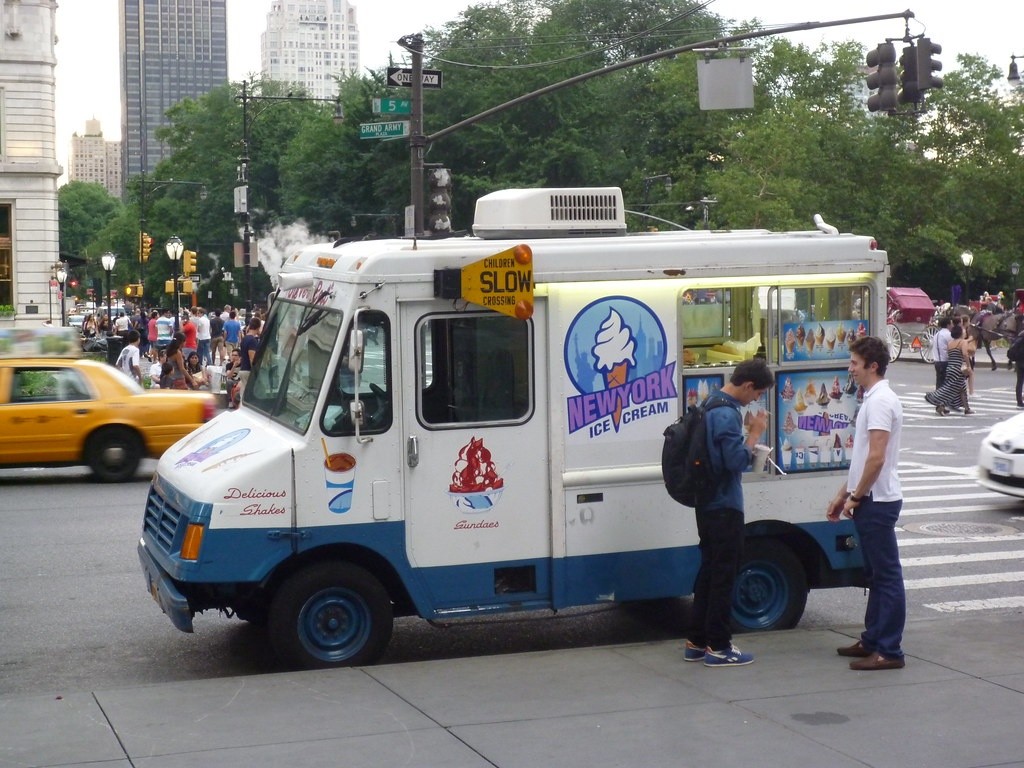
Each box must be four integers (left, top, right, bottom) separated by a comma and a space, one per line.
164, 233, 184, 339
961, 250, 974, 305
56, 268, 67, 326
1010, 262, 1020, 289
233, 80, 345, 326
136, 169, 207, 353
101, 251, 116, 336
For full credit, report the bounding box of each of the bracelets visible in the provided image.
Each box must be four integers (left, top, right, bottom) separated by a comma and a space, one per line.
850, 491, 860, 502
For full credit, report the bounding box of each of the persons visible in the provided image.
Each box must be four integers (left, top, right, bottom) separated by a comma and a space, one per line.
924, 315, 979, 417
684, 359, 774, 667
1016, 330, 1024, 407
83, 304, 267, 409
827, 337, 906, 671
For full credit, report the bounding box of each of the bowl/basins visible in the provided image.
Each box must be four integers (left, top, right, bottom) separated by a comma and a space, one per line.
444, 483, 508, 514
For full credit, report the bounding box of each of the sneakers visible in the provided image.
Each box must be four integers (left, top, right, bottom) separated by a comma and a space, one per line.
704, 644, 755, 665
685, 640, 708, 662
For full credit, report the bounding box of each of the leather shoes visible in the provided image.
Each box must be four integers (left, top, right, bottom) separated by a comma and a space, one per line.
837, 638, 871, 656
849, 647, 905, 671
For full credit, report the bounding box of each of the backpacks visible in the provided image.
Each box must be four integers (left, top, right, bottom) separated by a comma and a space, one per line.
661, 398, 742, 508
1008, 334, 1024, 361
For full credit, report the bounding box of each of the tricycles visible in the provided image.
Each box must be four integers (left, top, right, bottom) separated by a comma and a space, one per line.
887, 288, 942, 364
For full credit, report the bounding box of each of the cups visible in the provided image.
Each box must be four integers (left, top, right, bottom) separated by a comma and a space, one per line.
753, 445, 770, 473
323, 454, 355, 514
782, 447, 854, 465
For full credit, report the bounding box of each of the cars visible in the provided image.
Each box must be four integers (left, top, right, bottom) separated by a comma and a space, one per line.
1, 357, 217, 483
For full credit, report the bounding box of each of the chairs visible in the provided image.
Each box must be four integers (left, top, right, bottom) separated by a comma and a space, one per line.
436, 348, 514, 421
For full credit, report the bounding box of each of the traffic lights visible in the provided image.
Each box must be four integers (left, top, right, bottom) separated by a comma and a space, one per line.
126, 286, 131, 296
142, 235, 153, 261
184, 250, 197, 273
865, 43, 897, 112
897, 47, 923, 104
70, 281, 76, 287
918, 38, 943, 90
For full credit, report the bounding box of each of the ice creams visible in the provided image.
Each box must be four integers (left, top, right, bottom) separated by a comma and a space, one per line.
786, 322, 868, 355
782, 370, 865, 434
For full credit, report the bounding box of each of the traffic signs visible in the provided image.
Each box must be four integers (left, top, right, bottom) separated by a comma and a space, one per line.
387, 67, 443, 91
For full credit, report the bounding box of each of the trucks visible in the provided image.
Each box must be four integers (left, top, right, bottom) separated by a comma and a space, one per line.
134, 188, 891, 673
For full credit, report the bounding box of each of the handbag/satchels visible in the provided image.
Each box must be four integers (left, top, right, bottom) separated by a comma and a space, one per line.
967, 341, 977, 353
961, 362, 969, 377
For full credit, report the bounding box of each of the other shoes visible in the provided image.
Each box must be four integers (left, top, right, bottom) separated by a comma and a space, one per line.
965, 408, 977, 414
943, 410, 950, 413
141, 355, 144, 359
936, 406, 945, 416
